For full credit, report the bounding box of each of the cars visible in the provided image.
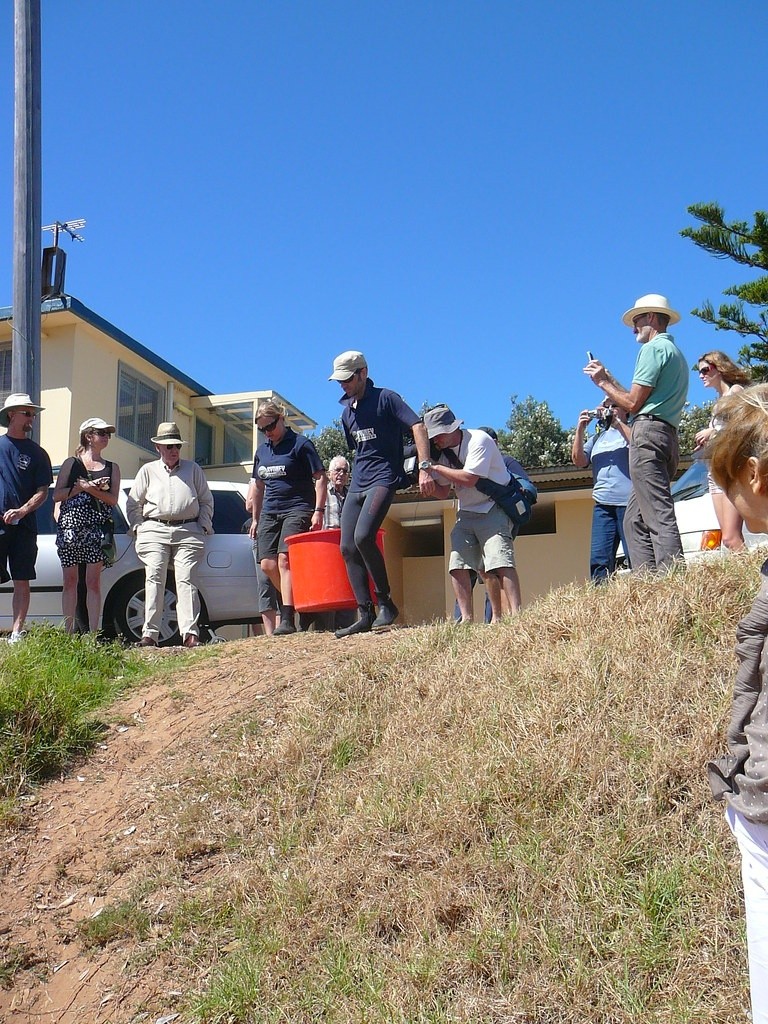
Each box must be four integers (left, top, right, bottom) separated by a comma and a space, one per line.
0, 475, 264, 647
614, 456, 768, 576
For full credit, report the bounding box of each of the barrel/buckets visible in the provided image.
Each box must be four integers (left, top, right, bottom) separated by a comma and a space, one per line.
283, 526, 387, 613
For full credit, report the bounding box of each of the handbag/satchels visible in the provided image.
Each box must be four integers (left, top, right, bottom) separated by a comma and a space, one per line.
99, 526, 117, 564
493, 474, 532, 526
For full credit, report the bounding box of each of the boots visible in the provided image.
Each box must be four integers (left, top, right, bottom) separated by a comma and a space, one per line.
371, 584, 399, 631
335, 600, 377, 638
273, 605, 298, 635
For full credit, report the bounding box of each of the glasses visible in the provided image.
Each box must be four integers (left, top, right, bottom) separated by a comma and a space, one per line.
633, 315, 646, 324
604, 404, 618, 408
91, 431, 110, 437
699, 365, 715, 375
158, 445, 182, 449
337, 374, 357, 383
427, 403, 447, 413
258, 417, 279, 433
13, 410, 38, 417
329, 468, 349, 475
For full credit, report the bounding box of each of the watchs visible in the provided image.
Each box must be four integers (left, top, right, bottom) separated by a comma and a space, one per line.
418, 461, 432, 470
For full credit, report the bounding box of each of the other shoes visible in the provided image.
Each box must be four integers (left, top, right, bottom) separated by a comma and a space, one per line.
134, 637, 157, 647
184, 634, 202, 647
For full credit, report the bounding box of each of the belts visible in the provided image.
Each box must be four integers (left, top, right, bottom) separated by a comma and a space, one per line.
148, 518, 198, 525
633, 414, 676, 429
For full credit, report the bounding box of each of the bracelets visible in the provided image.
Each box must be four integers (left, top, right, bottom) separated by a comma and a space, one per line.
314, 508, 325, 513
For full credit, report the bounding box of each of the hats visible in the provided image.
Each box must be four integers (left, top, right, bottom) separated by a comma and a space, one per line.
622, 294, 680, 327
601, 395, 610, 407
0, 393, 46, 427
328, 351, 367, 381
150, 422, 188, 445
79, 418, 116, 435
424, 408, 464, 439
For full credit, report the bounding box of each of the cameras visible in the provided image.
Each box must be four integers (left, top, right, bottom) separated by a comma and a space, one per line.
587, 410, 598, 418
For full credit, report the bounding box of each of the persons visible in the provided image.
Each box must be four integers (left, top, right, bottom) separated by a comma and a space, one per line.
328, 350, 435, 638
707, 376, 768, 1024
245, 400, 327, 635
572, 396, 632, 586
53, 418, 120, 640
310, 455, 357, 633
453, 426, 538, 622
694, 350, 754, 554
0, 392, 54, 643
424, 402, 522, 624
127, 421, 214, 647
582, 294, 689, 579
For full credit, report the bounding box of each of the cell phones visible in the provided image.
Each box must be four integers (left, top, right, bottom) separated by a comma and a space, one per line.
587, 350, 594, 362
